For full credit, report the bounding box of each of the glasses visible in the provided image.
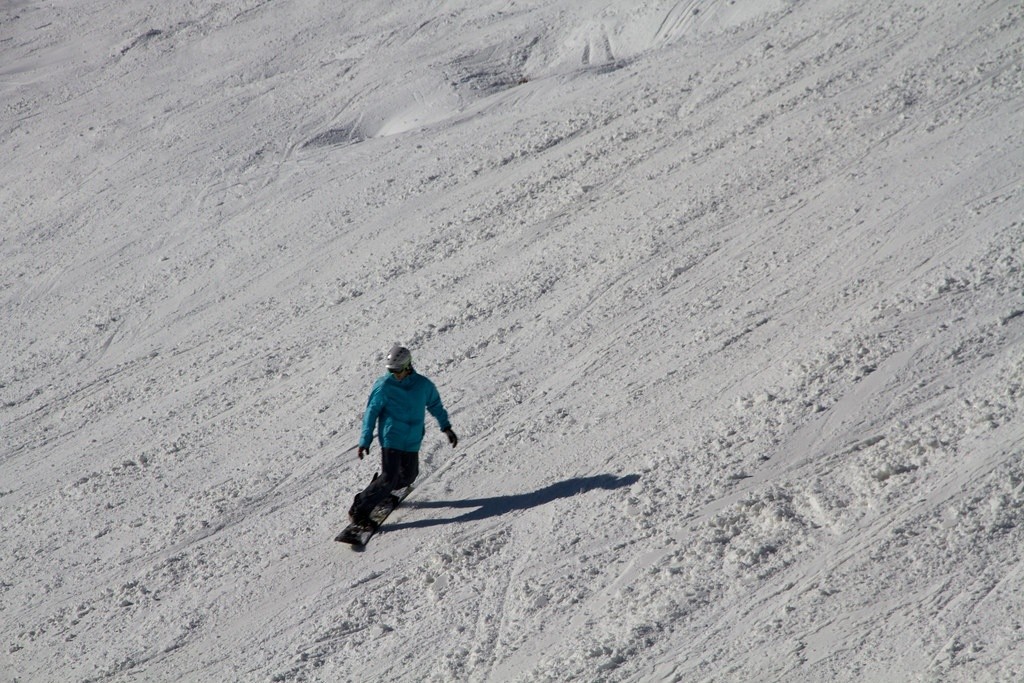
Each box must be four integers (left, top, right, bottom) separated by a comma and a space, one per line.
388, 367, 404, 374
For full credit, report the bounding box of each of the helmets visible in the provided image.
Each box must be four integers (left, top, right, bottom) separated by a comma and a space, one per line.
385, 346, 411, 370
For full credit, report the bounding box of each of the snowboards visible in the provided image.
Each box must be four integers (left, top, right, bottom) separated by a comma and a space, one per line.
333, 483, 412, 547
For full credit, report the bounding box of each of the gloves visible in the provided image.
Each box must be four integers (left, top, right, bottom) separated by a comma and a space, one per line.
358, 446, 369, 460
445, 427, 458, 448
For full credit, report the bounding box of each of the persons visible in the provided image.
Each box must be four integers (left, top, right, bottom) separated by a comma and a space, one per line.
349, 347, 458, 532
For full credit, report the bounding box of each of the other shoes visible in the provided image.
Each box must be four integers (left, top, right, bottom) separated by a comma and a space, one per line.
351, 518, 378, 530
378, 496, 400, 508
